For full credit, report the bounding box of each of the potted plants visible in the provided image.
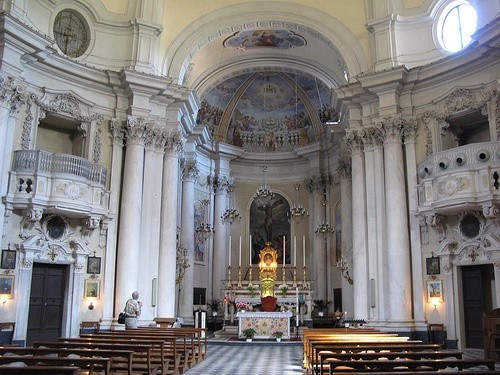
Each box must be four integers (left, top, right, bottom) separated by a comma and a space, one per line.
243, 328, 257, 342
209, 300, 220, 315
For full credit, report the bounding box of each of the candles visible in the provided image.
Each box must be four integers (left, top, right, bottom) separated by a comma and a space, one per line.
282, 234, 308, 267
227, 233, 252, 267
296, 288, 299, 326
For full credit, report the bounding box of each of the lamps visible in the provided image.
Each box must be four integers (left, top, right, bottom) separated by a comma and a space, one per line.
287, 203, 310, 226
228, 185, 236, 194
196, 223, 216, 242
254, 184, 276, 207
220, 207, 242, 225
176, 247, 191, 283
336, 255, 353, 285
314, 219, 335, 243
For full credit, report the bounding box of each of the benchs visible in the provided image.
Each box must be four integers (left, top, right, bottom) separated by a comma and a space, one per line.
0, 326, 208, 375
302, 327, 500, 375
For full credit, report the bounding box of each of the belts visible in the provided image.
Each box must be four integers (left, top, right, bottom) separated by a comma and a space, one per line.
126, 316, 136, 318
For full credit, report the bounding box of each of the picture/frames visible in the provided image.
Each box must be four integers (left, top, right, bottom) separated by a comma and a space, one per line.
0, 274, 15, 299
0, 249, 17, 269
87, 256, 101, 274
425, 257, 441, 275
427, 280, 443, 303
85, 279, 100, 300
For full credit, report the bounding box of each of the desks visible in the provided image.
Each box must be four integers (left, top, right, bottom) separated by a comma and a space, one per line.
238, 311, 292, 339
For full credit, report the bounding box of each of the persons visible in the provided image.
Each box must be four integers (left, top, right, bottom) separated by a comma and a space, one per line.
124, 291, 142, 330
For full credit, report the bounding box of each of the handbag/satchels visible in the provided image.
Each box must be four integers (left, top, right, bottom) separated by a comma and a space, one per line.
118, 313, 125, 324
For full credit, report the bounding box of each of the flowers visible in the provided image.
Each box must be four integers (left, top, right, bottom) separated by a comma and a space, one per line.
236, 301, 251, 310
248, 283, 259, 290
271, 329, 283, 338
279, 285, 289, 290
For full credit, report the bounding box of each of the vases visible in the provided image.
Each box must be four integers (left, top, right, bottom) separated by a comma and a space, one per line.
276, 338, 281, 342
283, 291, 287, 298
241, 310, 246, 312
250, 290, 255, 298
